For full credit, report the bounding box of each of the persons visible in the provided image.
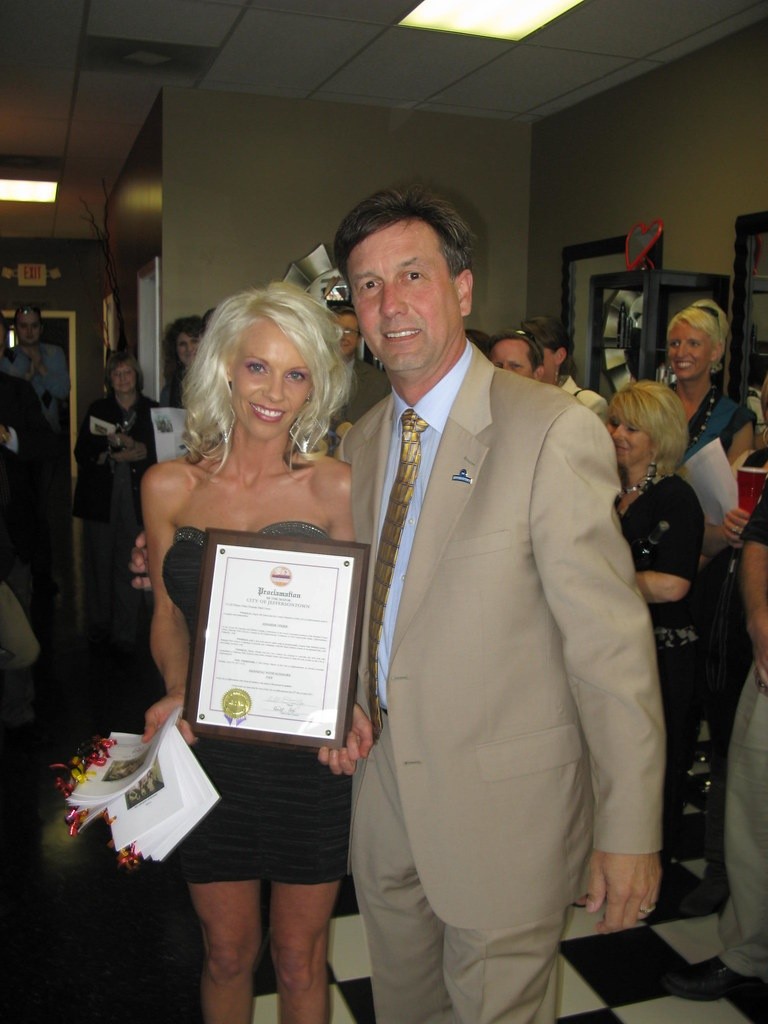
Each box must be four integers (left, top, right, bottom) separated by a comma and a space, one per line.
160, 317, 205, 407
606, 381, 705, 912
657, 473, 768, 1002
141, 280, 373, 1024
129, 185, 665, 1024
0, 302, 71, 745
462, 327, 545, 381
75, 349, 163, 664
662, 298, 757, 467
325, 300, 391, 455
519, 316, 608, 424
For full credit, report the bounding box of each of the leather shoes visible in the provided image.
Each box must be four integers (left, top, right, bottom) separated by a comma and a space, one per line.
667, 955, 768, 1002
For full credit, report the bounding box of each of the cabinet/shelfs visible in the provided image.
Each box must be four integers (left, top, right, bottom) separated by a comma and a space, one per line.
587, 271, 733, 399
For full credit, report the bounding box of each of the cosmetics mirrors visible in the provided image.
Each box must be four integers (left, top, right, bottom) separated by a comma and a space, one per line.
625, 220, 664, 270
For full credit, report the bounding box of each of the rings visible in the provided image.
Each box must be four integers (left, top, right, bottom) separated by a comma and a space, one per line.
640, 905, 656, 915
731, 526, 740, 533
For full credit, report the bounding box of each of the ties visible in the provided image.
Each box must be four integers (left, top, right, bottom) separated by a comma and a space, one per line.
367, 409, 429, 745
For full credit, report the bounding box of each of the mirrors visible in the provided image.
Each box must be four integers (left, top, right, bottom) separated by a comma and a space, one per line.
728, 212, 768, 407
562, 227, 665, 400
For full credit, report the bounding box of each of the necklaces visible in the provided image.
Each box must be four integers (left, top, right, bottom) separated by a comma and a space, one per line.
686, 386, 717, 450
121, 409, 137, 428
622, 476, 649, 493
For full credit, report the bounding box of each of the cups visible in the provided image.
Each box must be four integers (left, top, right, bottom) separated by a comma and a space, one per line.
736, 467, 767, 513
